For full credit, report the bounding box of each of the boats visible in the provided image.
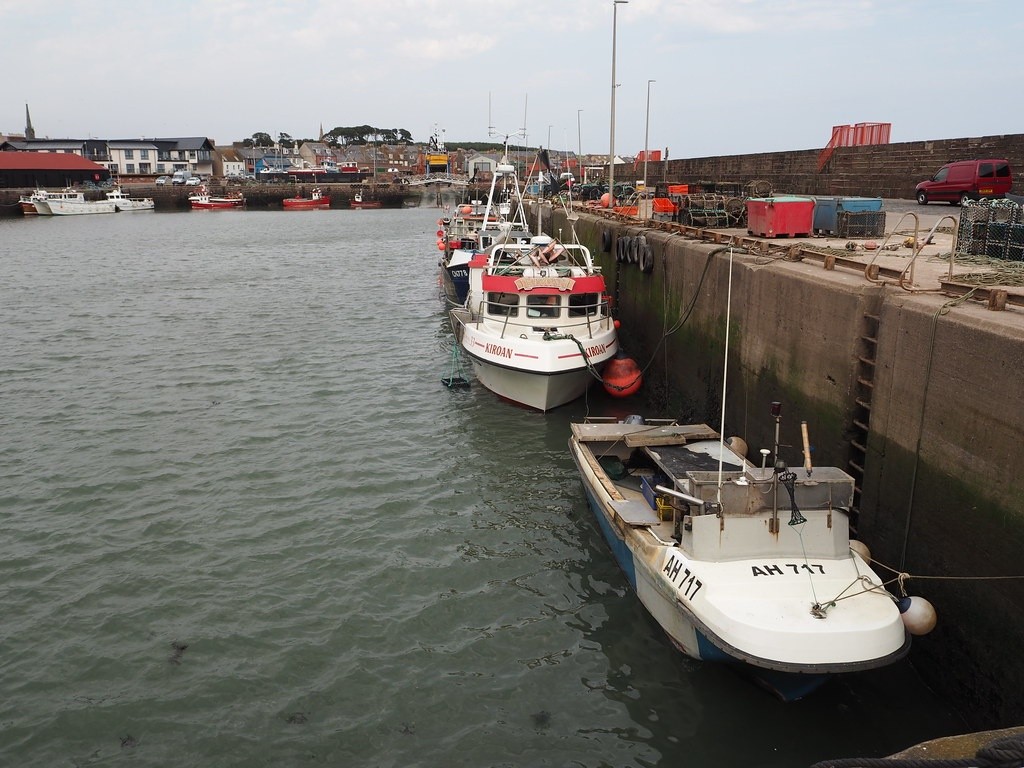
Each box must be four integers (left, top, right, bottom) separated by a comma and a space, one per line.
436, 161, 643, 417
281, 187, 332, 210
18, 167, 156, 216
349, 191, 382, 210
567, 244, 936, 704
187, 183, 247, 211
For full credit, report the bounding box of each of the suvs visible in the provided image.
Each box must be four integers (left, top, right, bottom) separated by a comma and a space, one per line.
913, 159, 1013, 208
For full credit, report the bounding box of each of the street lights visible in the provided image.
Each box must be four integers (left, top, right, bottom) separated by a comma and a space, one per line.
578, 107, 586, 184
642, 78, 657, 196
609, 0, 630, 211
548, 124, 555, 156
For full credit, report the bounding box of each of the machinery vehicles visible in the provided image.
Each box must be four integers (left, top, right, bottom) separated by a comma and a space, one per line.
426, 135, 448, 172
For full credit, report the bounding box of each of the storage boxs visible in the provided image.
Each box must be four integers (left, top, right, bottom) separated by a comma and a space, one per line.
655, 497, 674, 522
641, 475, 674, 510
613, 180, 884, 237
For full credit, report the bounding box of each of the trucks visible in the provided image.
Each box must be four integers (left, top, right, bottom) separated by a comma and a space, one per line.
172, 171, 191, 184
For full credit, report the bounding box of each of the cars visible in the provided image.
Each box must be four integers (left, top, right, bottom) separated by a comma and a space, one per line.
155, 175, 172, 186
185, 176, 201, 186
